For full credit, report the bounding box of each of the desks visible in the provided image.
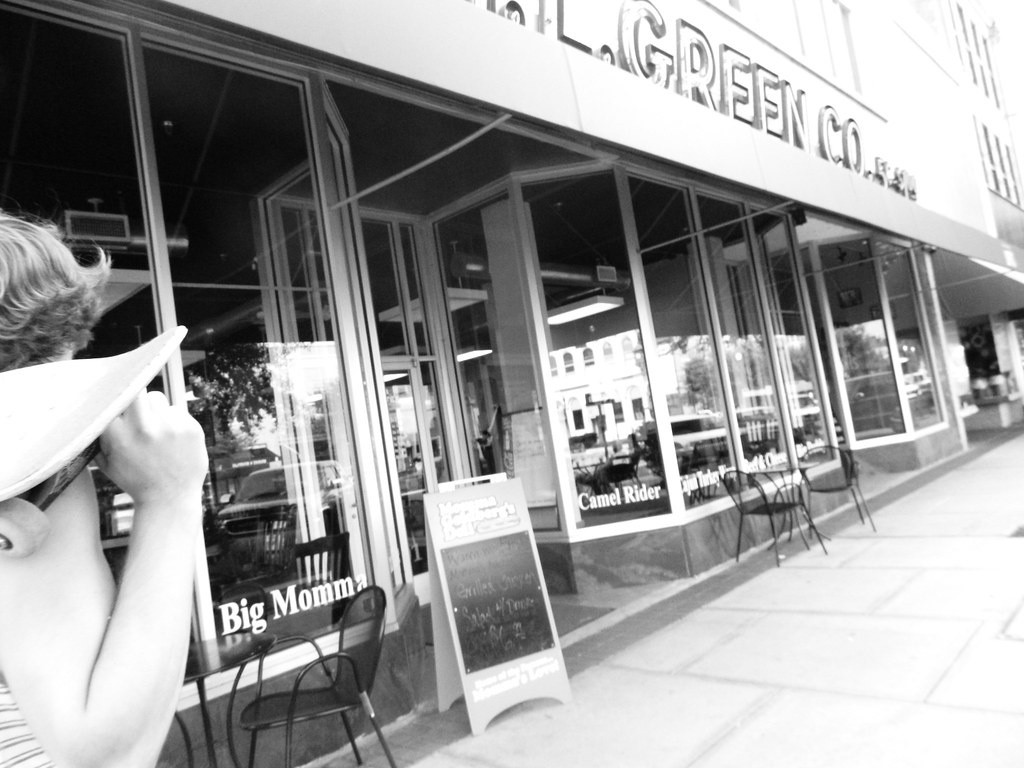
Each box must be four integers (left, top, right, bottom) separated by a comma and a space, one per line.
174, 630, 277, 768
746, 460, 831, 550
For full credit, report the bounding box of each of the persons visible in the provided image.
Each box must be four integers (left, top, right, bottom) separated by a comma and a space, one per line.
0, 214, 210, 768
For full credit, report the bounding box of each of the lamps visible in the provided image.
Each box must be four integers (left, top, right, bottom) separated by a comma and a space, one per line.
129, 223, 190, 254
544, 287, 624, 326
456, 343, 493, 362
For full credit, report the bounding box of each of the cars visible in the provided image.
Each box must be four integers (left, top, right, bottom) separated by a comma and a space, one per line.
570, 431, 637, 498
734, 392, 823, 450
903, 374, 932, 411
393, 433, 447, 490
644, 411, 728, 470
832, 369, 908, 433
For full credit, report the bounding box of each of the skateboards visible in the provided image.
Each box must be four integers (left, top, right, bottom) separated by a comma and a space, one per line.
1, 325, 191, 558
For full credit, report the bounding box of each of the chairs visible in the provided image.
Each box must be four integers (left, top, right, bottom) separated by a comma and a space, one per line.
216, 531, 400, 768
724, 445, 876, 567
573, 442, 649, 507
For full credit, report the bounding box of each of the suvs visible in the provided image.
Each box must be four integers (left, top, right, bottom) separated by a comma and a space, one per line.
214, 458, 343, 550
